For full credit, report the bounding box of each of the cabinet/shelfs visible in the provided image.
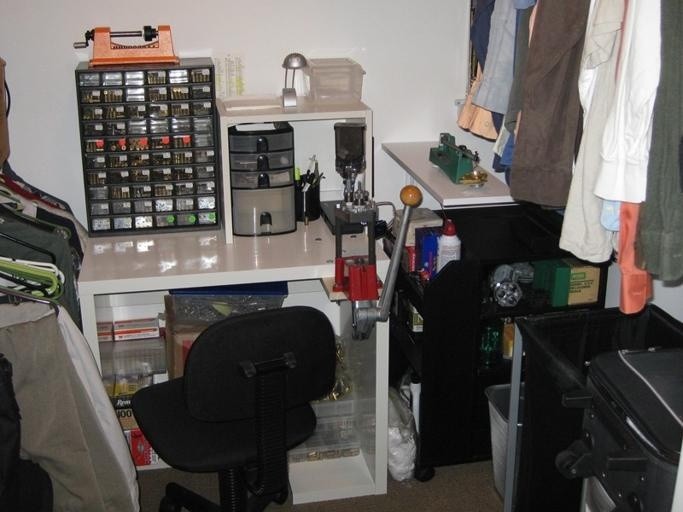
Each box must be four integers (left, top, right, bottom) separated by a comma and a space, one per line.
71, 97, 391, 506
382, 138, 619, 484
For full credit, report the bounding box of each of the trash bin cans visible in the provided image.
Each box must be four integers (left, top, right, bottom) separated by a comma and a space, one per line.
485, 381, 524, 506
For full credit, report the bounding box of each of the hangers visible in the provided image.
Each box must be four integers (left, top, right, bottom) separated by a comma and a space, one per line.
2, 164, 71, 315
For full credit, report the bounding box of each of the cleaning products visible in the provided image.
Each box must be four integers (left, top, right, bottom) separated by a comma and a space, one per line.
437, 221, 462, 270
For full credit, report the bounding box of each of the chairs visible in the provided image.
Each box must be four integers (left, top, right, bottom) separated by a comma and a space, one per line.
124, 305, 339, 512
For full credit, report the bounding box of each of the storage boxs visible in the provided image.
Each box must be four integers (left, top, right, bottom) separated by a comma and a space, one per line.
97, 304, 361, 464
304, 57, 367, 108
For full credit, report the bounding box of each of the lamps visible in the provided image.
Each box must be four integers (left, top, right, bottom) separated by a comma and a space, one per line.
278, 52, 308, 108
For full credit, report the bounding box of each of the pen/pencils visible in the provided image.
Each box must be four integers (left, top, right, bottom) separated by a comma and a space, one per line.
294, 155, 326, 192
